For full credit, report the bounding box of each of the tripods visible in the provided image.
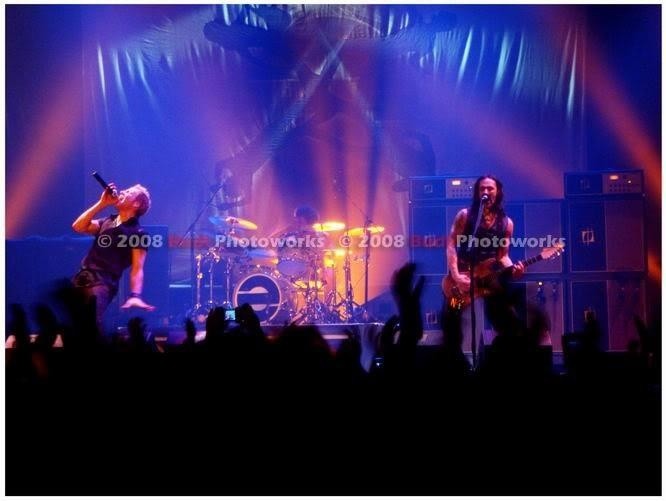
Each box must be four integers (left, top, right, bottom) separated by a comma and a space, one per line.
175, 179, 382, 329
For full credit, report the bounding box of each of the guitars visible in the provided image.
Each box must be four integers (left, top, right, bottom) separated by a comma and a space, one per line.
442, 243, 566, 310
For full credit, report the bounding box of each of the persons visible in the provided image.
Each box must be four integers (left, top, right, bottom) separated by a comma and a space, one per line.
268, 206, 327, 309
441, 174, 525, 351
72, 183, 155, 335
6, 262, 661, 497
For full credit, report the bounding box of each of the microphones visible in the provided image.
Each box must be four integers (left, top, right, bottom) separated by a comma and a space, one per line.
90, 169, 119, 199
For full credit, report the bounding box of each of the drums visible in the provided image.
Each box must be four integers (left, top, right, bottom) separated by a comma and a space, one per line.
233, 271, 298, 327
291, 266, 328, 290
211, 234, 251, 257
275, 240, 310, 278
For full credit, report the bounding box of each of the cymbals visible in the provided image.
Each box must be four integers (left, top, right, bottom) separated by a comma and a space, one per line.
344, 224, 384, 239
312, 222, 348, 233
209, 216, 258, 231
